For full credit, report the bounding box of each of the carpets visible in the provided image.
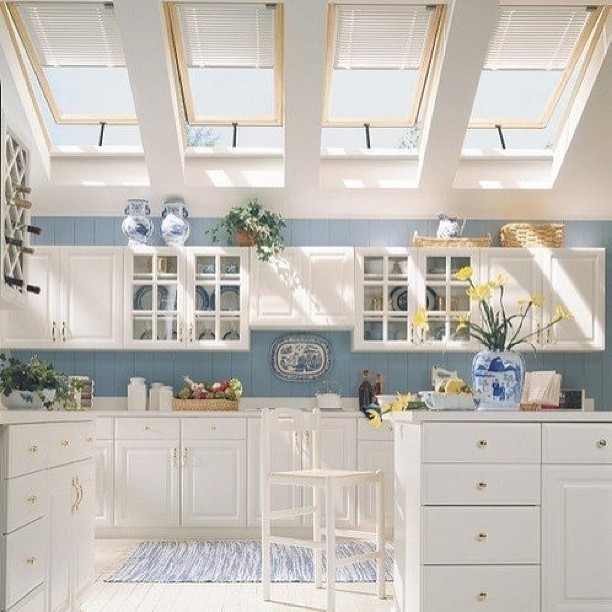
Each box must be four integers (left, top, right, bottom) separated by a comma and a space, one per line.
104, 538, 391, 582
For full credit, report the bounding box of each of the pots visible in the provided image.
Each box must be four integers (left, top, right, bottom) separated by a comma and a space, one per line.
398, 260, 411, 274
437, 294, 459, 314
372, 293, 394, 314
367, 257, 396, 274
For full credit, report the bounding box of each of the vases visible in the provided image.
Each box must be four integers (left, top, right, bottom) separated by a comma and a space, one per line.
122, 198, 155, 247
472, 350, 525, 411
160, 202, 191, 247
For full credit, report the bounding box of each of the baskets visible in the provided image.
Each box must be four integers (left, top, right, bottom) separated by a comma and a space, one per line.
170, 397, 239, 411
412, 230, 493, 247
418, 388, 482, 412
499, 222, 565, 247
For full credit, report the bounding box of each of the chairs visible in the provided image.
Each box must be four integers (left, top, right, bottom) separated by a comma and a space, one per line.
542, 411, 611, 612
260, 406, 386, 612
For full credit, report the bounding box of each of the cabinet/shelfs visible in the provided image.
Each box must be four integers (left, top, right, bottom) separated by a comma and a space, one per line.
381, 411, 541, 612
264, 412, 347, 526
350, 247, 480, 353
357, 412, 394, 528
1, 411, 96, 612
95, 412, 114, 538
0, 246, 123, 350
4, 125, 32, 303
115, 412, 246, 538
125, 246, 249, 351
250, 247, 354, 331
481, 247, 608, 353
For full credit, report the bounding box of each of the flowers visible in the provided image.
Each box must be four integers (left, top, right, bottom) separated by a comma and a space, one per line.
413, 267, 574, 354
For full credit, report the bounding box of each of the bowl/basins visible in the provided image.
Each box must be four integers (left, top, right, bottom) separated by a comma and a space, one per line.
373, 394, 410, 411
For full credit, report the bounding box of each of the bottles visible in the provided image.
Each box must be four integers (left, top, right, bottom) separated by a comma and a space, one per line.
374, 374, 384, 410
148, 382, 162, 410
127, 377, 148, 412
357, 371, 373, 412
159, 385, 172, 411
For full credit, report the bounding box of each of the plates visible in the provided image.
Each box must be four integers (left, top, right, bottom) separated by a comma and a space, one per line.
199, 332, 215, 341
268, 334, 334, 382
164, 332, 179, 341
210, 286, 240, 313
133, 285, 171, 313
174, 285, 209, 317
224, 332, 241, 340
139, 330, 159, 341
388, 283, 437, 320
430, 266, 459, 274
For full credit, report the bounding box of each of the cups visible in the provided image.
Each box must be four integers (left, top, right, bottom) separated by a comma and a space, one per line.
202, 264, 214, 273
226, 264, 238, 274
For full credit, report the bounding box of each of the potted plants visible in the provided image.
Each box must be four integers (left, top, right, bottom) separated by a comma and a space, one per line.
0, 353, 85, 411
205, 191, 288, 262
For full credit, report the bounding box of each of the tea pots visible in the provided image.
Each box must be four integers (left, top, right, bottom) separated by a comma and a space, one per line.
314, 386, 341, 409
